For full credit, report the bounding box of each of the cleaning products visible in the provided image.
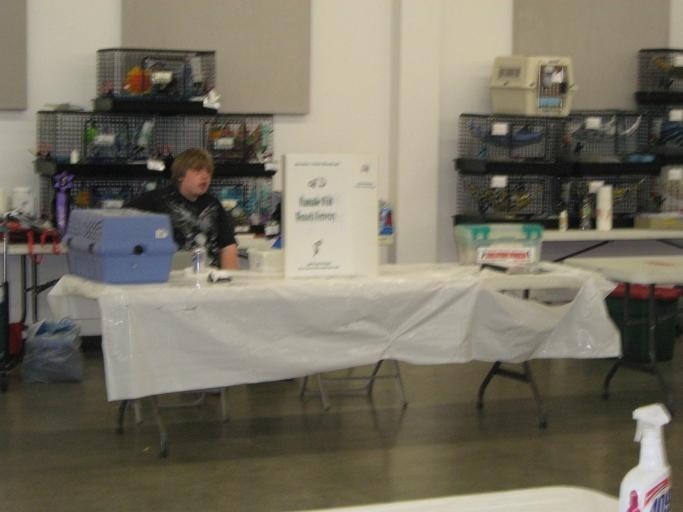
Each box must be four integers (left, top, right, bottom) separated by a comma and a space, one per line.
619, 403, 672, 510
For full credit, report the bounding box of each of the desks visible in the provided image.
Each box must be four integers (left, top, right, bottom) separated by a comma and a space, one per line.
62, 261, 597, 458
544, 229, 683, 262
295, 484, 619, 512
563, 254, 683, 416
0, 233, 393, 325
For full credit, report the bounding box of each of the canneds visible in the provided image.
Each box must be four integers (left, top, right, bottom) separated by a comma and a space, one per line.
192, 248, 208, 274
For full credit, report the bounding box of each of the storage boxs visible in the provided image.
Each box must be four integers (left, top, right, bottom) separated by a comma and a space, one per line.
452, 224, 544, 264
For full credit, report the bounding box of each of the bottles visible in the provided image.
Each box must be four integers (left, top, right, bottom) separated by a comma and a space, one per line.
580, 200, 591, 230
558, 209, 568, 231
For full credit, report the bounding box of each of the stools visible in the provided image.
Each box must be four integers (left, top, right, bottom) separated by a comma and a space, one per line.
127, 386, 230, 425
300, 361, 409, 412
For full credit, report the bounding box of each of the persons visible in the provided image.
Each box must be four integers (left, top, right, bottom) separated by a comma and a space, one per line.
118, 145, 241, 396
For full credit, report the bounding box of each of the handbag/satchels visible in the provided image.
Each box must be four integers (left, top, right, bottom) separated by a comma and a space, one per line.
0, 209, 61, 244
